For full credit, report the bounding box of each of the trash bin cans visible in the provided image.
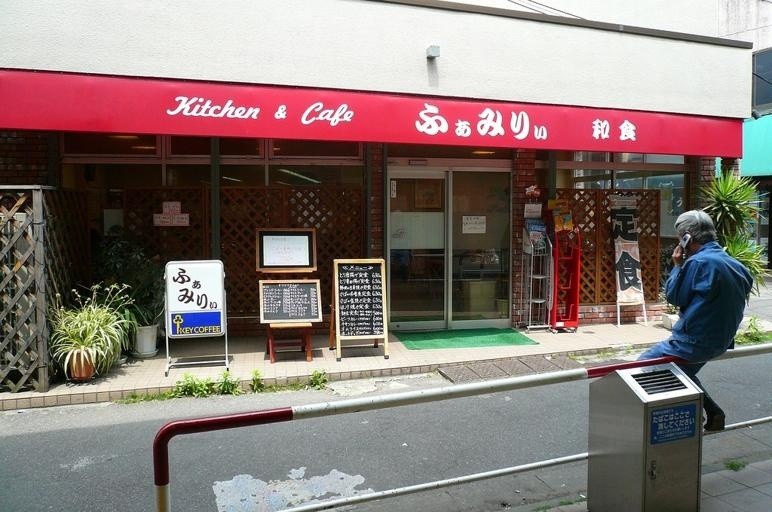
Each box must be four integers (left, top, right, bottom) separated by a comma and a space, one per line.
589, 362, 705, 512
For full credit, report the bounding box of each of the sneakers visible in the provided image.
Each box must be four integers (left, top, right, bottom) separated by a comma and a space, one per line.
704, 407, 725, 431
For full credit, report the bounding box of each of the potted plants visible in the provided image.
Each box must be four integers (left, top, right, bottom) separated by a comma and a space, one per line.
656, 286, 680, 330
47, 235, 165, 383
495, 279, 509, 317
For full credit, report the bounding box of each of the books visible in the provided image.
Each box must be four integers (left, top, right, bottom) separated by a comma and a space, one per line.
524, 217, 547, 248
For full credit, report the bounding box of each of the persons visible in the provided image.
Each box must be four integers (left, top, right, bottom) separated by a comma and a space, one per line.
634, 209, 755, 431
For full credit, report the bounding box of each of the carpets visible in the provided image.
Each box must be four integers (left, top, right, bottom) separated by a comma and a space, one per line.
389, 314, 540, 351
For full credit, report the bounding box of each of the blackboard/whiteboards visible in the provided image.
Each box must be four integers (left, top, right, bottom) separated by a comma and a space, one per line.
258, 279, 323, 324
334, 261, 388, 338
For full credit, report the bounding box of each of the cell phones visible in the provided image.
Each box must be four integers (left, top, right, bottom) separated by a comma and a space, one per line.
680, 234, 691, 249
